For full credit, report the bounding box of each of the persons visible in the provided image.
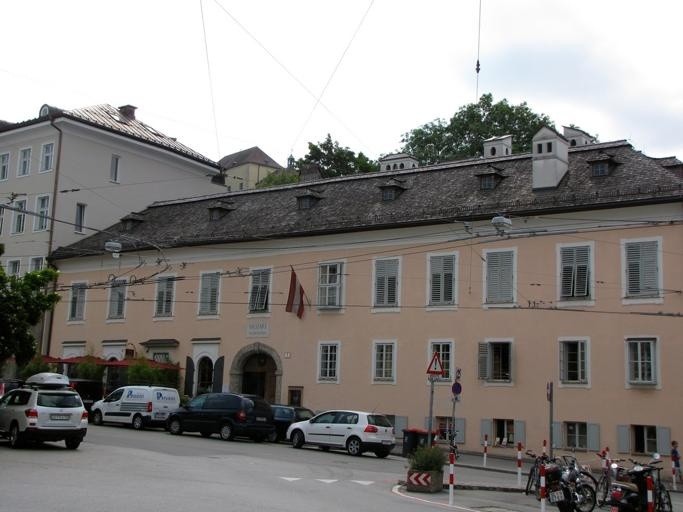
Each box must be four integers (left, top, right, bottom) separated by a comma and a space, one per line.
669, 440, 683, 483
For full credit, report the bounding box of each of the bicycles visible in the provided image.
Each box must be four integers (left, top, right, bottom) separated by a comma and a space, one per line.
525, 449, 670, 512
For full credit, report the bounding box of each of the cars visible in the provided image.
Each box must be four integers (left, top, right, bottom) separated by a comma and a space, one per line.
270, 404, 395, 459
163, 394, 277, 446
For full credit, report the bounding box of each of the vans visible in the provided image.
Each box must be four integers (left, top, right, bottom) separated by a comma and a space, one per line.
68, 377, 102, 421
89, 386, 179, 430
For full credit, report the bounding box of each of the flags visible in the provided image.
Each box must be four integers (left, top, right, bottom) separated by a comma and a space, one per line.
284, 270, 304, 319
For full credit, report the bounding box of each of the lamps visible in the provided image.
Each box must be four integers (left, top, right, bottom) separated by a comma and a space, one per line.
104, 235, 122, 252
491, 217, 512, 233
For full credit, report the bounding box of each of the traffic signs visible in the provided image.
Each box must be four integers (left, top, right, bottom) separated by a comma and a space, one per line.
409, 473, 431, 486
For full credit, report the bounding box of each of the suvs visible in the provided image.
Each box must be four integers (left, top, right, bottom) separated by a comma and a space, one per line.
0, 372, 88, 449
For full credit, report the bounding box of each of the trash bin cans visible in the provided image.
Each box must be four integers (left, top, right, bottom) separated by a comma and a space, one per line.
402, 427, 436, 459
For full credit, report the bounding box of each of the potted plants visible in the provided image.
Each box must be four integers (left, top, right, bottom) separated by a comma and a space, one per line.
407, 444, 448, 493
